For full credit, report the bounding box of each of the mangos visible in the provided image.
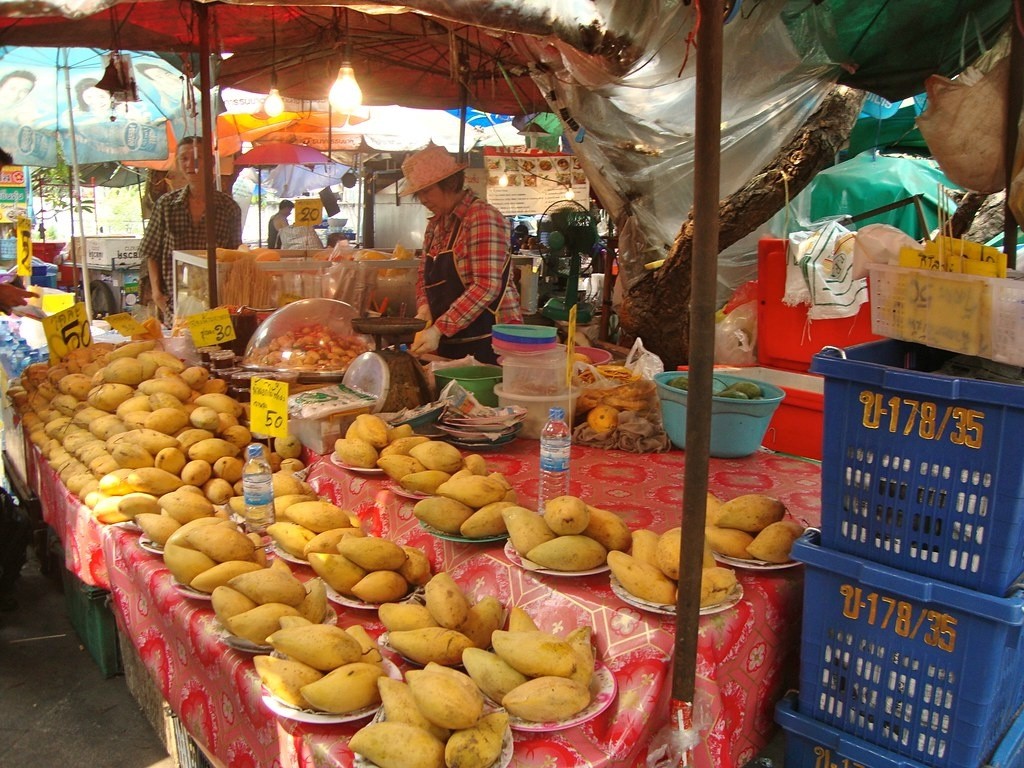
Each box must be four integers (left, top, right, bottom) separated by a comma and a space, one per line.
668, 377, 761, 399
587, 405, 621, 436
134, 471, 594, 768
334, 414, 805, 609
6, 340, 306, 526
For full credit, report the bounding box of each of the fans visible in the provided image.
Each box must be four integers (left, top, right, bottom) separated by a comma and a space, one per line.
536, 199, 598, 324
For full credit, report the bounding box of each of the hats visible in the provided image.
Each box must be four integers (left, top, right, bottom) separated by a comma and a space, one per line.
398, 145, 469, 197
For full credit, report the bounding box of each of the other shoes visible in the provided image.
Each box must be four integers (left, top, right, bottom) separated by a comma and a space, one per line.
0, 592, 19, 611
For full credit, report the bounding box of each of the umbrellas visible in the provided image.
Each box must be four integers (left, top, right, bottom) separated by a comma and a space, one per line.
0, 1, 624, 327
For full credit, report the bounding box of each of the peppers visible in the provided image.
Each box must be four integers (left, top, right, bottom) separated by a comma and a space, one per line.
288, 322, 333, 356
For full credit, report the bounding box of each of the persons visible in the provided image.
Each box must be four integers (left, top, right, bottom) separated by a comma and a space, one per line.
75, 77, 113, 113
138, 136, 242, 329
399, 146, 524, 366
134, 63, 179, 100
0, 70, 38, 121
0, 283, 41, 316
268, 199, 294, 249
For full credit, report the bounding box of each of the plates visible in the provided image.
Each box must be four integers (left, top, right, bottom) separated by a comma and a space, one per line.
136, 450, 805, 768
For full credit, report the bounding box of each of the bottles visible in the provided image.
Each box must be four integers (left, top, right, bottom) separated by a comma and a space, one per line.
241, 443, 275, 534
536, 406, 572, 517
0, 319, 50, 381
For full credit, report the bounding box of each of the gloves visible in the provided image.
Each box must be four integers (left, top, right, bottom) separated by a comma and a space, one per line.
414, 306, 432, 329
408, 325, 442, 354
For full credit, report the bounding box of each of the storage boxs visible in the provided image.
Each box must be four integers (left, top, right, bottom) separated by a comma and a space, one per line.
678, 238, 1024, 768
31, 236, 143, 288
66, 577, 199, 768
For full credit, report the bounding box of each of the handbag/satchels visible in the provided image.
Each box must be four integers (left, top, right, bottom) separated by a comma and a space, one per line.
0, 487, 33, 592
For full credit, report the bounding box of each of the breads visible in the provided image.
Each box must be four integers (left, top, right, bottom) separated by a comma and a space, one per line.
355, 246, 416, 278
209, 247, 280, 306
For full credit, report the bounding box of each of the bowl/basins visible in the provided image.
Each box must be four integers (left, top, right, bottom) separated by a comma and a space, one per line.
654, 371, 786, 458
431, 323, 612, 440
189, 345, 300, 403
327, 219, 347, 227
